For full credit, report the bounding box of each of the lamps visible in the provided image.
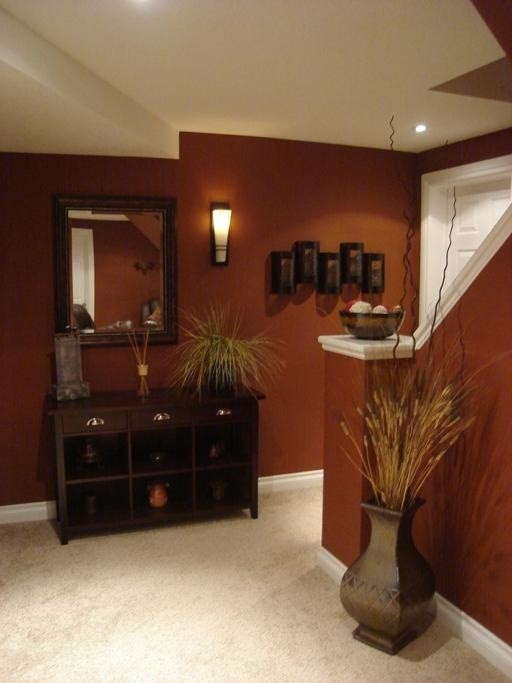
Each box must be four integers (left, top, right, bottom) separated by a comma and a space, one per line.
211, 208, 232, 264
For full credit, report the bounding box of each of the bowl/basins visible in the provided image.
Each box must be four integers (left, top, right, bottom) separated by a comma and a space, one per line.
338, 310, 404, 340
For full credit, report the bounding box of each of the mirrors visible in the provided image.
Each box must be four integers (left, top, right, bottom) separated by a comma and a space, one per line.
52, 193, 180, 347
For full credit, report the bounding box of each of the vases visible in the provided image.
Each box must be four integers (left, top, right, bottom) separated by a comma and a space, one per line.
341, 497, 439, 656
208, 363, 234, 397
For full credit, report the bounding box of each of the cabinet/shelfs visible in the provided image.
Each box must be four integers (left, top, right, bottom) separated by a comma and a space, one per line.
47, 383, 265, 546
